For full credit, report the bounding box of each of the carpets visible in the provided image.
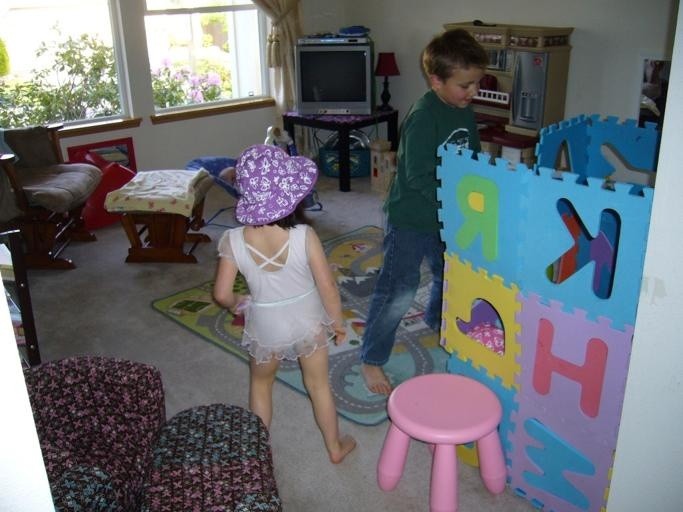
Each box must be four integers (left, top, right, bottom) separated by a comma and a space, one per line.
151, 225, 450, 426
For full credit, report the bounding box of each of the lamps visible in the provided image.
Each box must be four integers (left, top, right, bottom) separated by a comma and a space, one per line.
374, 52, 400, 111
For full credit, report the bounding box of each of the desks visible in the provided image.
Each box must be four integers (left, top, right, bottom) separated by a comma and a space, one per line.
283, 107, 399, 192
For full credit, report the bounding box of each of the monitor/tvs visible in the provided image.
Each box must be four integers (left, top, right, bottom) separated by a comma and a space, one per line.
294, 42, 372, 116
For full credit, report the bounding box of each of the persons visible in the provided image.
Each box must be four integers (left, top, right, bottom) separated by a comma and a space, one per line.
360, 28, 490, 395
212, 143, 356, 464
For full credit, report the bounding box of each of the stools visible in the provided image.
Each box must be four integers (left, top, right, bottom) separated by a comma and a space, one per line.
378, 372, 507, 512
138, 404, 284, 511
104, 166, 215, 263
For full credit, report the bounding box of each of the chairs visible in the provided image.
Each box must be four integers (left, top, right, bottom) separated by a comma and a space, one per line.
0, 123, 104, 270
19, 355, 165, 511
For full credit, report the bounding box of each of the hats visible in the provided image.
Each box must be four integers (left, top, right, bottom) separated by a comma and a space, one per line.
225, 143, 322, 227
